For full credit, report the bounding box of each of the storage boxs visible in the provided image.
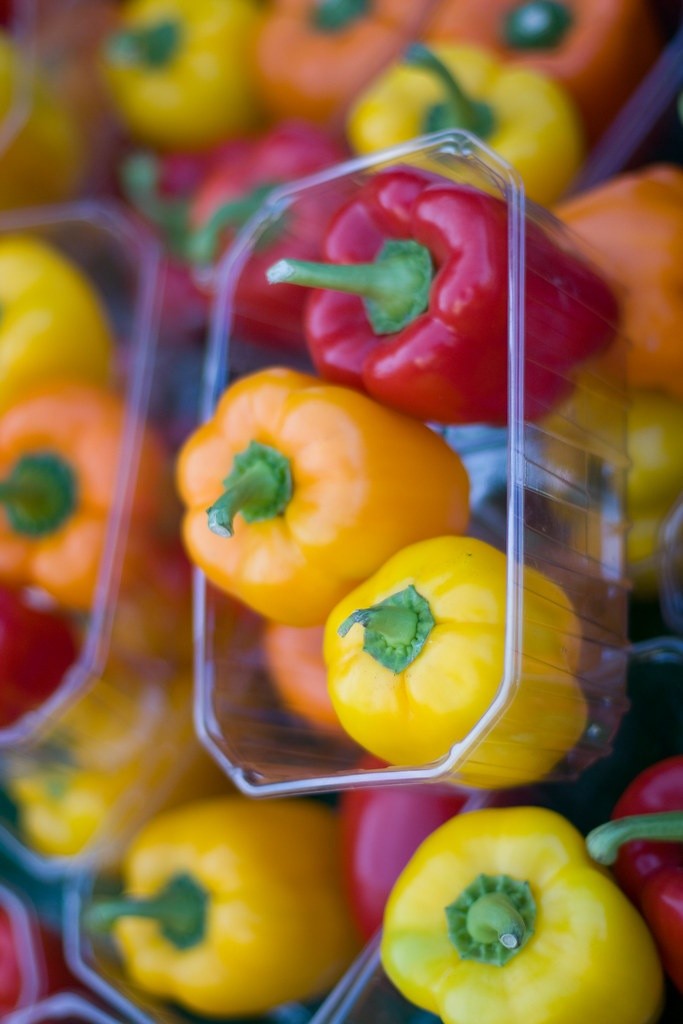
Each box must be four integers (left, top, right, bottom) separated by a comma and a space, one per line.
0, 128, 683, 1024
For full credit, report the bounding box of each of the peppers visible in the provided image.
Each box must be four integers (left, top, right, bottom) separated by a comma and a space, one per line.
0, 0, 683, 1024
